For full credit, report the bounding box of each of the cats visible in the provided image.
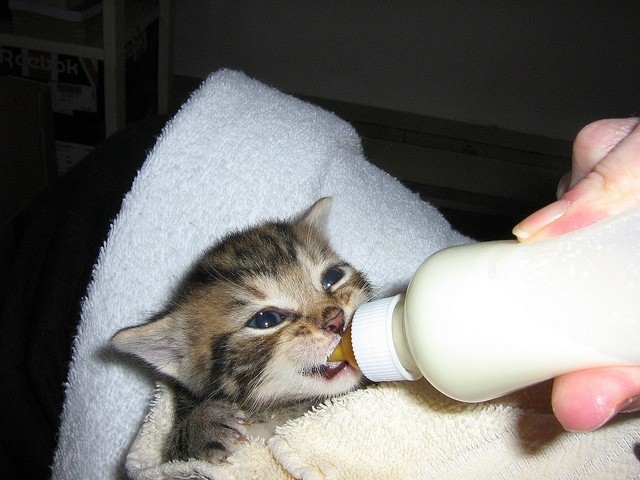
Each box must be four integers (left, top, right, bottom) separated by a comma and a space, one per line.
108, 196, 400, 467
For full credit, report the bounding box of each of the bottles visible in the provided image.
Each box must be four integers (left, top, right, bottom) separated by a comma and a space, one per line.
326, 206, 640, 405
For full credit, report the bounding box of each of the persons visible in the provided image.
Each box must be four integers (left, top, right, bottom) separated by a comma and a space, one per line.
511, 118, 640, 433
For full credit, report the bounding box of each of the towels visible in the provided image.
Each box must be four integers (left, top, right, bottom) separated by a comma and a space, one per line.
122, 376, 638, 480
48, 68, 474, 478
